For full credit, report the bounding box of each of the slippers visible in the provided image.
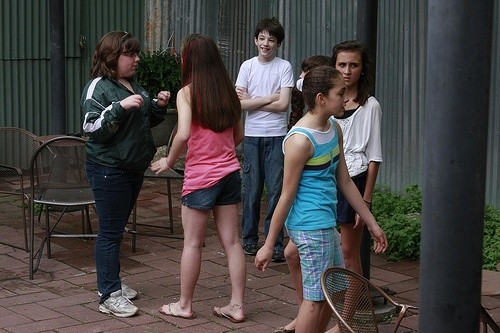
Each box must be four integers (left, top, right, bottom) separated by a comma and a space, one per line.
213, 306, 245, 322
157, 302, 197, 320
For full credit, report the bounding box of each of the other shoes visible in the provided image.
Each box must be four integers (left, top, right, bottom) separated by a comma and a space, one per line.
275, 326, 296, 333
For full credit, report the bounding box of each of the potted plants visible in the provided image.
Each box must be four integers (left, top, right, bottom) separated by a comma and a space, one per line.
132, 50, 183, 143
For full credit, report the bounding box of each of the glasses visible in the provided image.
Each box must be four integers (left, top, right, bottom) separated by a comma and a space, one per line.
121, 49, 141, 57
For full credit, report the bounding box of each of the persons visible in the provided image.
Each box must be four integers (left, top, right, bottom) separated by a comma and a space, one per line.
79, 30, 171, 317
298, 56, 333, 79
253, 64, 391, 333
274, 40, 383, 333
234, 16, 295, 263
149, 32, 246, 323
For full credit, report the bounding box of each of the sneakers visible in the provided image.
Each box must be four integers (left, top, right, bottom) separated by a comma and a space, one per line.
97, 284, 137, 300
244, 243, 258, 255
272, 248, 287, 262
98, 289, 140, 317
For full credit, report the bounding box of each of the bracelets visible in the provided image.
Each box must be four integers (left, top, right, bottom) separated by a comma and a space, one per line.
165, 157, 174, 169
363, 200, 372, 205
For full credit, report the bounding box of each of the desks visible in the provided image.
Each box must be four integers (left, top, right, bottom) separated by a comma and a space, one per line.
481, 271, 500, 296
31, 132, 98, 239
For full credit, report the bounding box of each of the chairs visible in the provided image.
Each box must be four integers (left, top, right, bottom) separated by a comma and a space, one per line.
124, 120, 206, 252
320, 266, 419, 333
0, 127, 54, 255
29, 136, 99, 279
